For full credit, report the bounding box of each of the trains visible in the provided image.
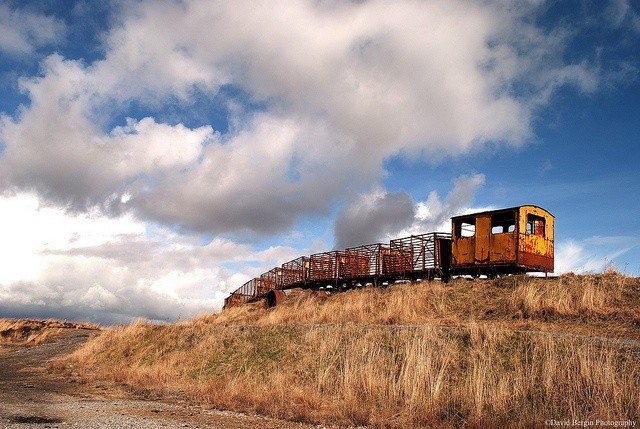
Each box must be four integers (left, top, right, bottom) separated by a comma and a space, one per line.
224, 205, 555, 310
